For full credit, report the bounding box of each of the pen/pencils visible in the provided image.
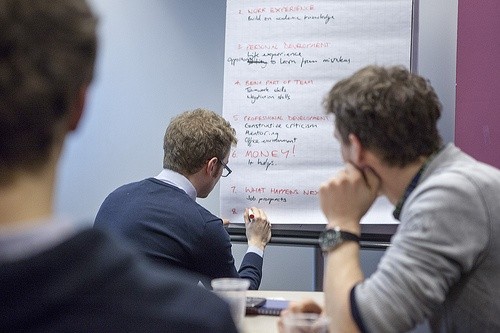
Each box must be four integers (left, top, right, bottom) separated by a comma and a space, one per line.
248, 208, 255, 219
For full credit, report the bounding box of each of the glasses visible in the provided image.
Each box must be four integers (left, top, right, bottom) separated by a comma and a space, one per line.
208, 157, 232, 178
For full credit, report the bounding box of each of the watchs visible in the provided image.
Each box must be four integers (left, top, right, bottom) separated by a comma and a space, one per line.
318, 226, 360, 257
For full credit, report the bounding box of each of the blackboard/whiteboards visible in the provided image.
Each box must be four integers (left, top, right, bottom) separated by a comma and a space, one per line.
221, 0, 419, 249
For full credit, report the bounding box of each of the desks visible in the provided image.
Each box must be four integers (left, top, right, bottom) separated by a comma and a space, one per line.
212, 291, 326, 332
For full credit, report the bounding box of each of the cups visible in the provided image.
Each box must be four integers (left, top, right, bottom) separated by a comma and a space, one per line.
282, 313, 326, 333
211, 278, 250, 329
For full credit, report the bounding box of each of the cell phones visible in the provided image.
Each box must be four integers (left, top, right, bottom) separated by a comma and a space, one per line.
246, 296, 266, 309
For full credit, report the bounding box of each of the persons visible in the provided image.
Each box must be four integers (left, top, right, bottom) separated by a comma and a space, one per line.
93, 108, 272, 291
0, 0, 237, 333
277, 64, 500, 333
